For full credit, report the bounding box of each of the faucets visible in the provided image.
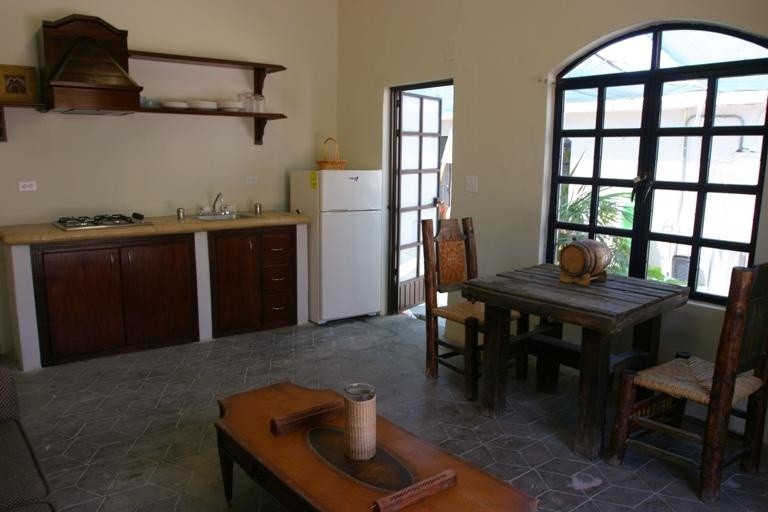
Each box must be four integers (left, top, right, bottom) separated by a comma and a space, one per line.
212, 191, 222, 212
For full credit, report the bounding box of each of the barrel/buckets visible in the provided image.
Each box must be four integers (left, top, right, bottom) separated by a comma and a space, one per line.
560, 238, 613, 276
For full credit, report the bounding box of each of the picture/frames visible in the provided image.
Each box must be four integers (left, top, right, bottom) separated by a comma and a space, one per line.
0, 64, 41, 104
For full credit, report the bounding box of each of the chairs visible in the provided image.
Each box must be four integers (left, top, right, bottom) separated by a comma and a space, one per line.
608, 264, 768, 504
0, 365, 61, 511
422, 218, 533, 399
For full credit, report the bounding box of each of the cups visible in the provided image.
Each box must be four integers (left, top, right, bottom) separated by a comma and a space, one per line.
238, 91, 267, 113
345, 383, 378, 463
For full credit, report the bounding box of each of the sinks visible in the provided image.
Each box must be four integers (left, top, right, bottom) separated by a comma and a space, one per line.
195, 212, 247, 221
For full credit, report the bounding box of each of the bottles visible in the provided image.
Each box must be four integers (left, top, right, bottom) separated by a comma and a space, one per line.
254, 203, 261, 215
176, 208, 184, 220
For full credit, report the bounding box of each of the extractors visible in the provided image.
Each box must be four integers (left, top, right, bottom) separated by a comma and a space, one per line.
34, 37, 142, 114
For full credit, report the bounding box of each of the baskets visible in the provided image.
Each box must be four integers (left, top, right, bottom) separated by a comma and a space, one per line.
315, 137, 348, 170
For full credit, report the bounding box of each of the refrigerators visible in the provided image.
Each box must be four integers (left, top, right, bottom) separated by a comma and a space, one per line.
287, 168, 381, 324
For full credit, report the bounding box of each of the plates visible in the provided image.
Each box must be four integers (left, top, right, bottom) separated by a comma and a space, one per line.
159, 99, 244, 113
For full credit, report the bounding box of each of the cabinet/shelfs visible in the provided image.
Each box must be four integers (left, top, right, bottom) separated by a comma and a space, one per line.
0, 11, 289, 148
211, 225, 296, 340
30, 232, 200, 368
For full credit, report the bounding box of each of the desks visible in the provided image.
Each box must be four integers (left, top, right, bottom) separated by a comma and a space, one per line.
461, 261, 690, 460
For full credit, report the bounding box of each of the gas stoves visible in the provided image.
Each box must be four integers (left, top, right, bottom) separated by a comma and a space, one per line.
53, 213, 148, 232
